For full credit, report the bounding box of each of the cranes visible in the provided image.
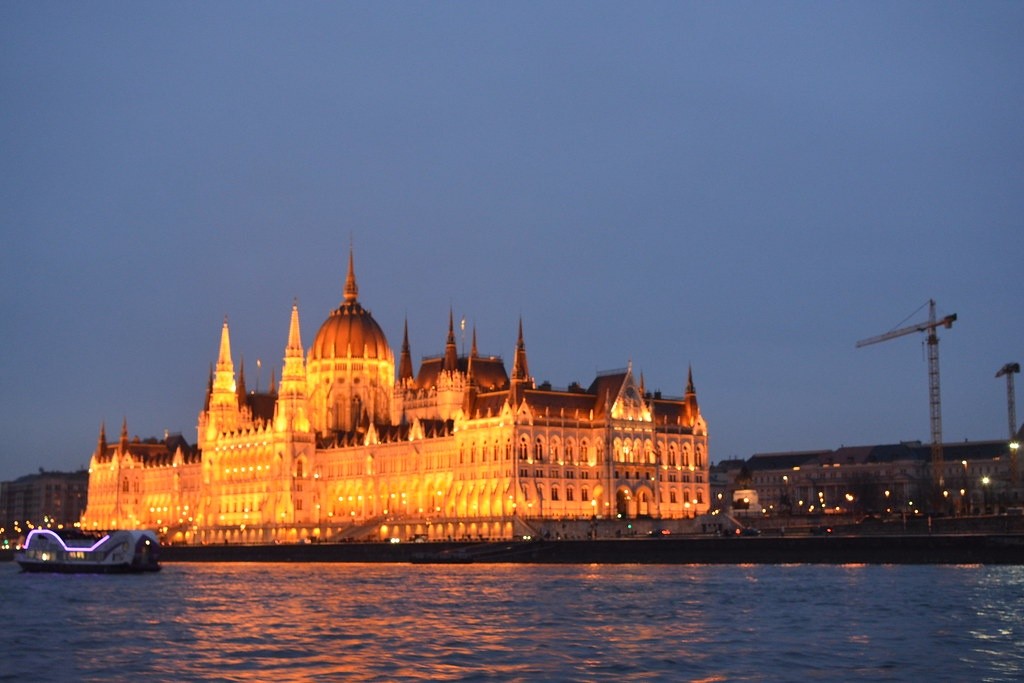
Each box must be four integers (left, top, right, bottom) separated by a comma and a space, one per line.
996, 362, 1020, 482
857, 298, 958, 462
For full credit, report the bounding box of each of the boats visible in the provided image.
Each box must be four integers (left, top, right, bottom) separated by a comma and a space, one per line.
14, 529, 162, 573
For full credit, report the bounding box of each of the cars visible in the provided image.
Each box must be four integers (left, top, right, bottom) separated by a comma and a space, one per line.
739, 527, 761, 536
648, 528, 670, 537
